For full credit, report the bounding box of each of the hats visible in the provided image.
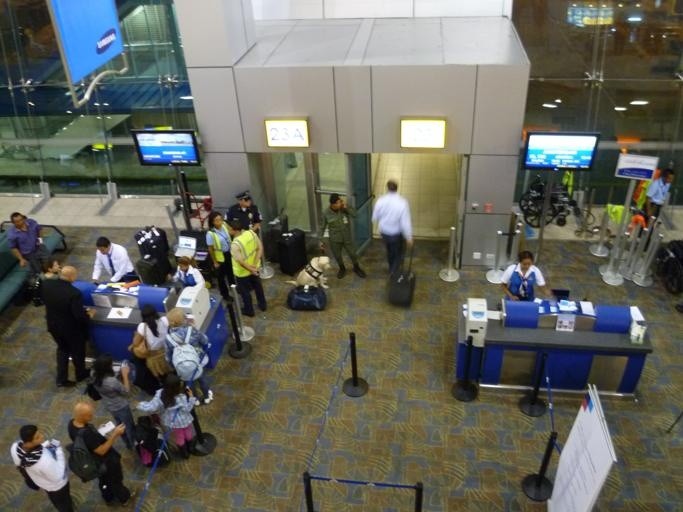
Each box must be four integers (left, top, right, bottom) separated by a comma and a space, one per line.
236, 190, 250, 200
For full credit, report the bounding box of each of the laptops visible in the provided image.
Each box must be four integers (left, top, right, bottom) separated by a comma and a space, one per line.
174, 235, 198, 257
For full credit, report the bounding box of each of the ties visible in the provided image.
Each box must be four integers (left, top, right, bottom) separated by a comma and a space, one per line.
108, 253, 116, 277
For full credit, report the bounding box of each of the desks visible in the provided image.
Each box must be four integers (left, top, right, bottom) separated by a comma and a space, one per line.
74, 279, 228, 370
457, 298, 652, 399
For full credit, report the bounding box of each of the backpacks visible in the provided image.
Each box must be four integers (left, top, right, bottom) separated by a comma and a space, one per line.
167, 326, 204, 381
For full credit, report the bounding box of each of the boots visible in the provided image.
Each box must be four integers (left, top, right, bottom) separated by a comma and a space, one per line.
354, 263, 365, 278
337, 264, 345, 279
187, 440, 195, 453
180, 444, 188, 459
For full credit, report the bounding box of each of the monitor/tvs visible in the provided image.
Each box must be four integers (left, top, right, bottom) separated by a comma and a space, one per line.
522, 131, 601, 172
129, 128, 202, 167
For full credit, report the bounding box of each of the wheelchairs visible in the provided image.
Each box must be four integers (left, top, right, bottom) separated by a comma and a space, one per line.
520, 173, 581, 229
657, 238, 682, 296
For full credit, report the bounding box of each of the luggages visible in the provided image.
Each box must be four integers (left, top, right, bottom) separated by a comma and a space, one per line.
388, 245, 416, 307
145, 225, 169, 251
278, 228, 306, 275
135, 230, 172, 268
29, 276, 45, 307
269, 214, 288, 263
137, 255, 165, 285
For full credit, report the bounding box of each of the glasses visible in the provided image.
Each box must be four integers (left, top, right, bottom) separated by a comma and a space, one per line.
245, 197, 250, 201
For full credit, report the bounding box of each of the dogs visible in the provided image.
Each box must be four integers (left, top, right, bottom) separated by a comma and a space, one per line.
283, 256, 331, 290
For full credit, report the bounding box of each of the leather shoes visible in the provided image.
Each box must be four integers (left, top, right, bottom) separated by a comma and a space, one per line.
57, 381, 76, 387
77, 368, 91, 381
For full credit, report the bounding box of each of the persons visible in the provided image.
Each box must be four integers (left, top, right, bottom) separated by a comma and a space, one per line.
90, 351, 136, 449
8, 213, 51, 272
206, 212, 235, 303
10, 425, 74, 512
167, 256, 205, 288
636, 170, 675, 253
227, 191, 262, 234
128, 304, 212, 405
91, 237, 134, 283
136, 374, 196, 458
501, 251, 553, 301
67, 402, 136, 509
228, 219, 267, 316
372, 180, 412, 276
318, 192, 367, 279
32, 259, 96, 386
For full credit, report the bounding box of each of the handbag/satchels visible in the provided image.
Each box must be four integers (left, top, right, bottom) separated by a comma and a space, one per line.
134, 414, 168, 466
69, 428, 108, 482
288, 285, 326, 309
146, 349, 167, 377
20, 455, 39, 490
135, 416, 158, 451
84, 378, 102, 401
133, 336, 148, 359
